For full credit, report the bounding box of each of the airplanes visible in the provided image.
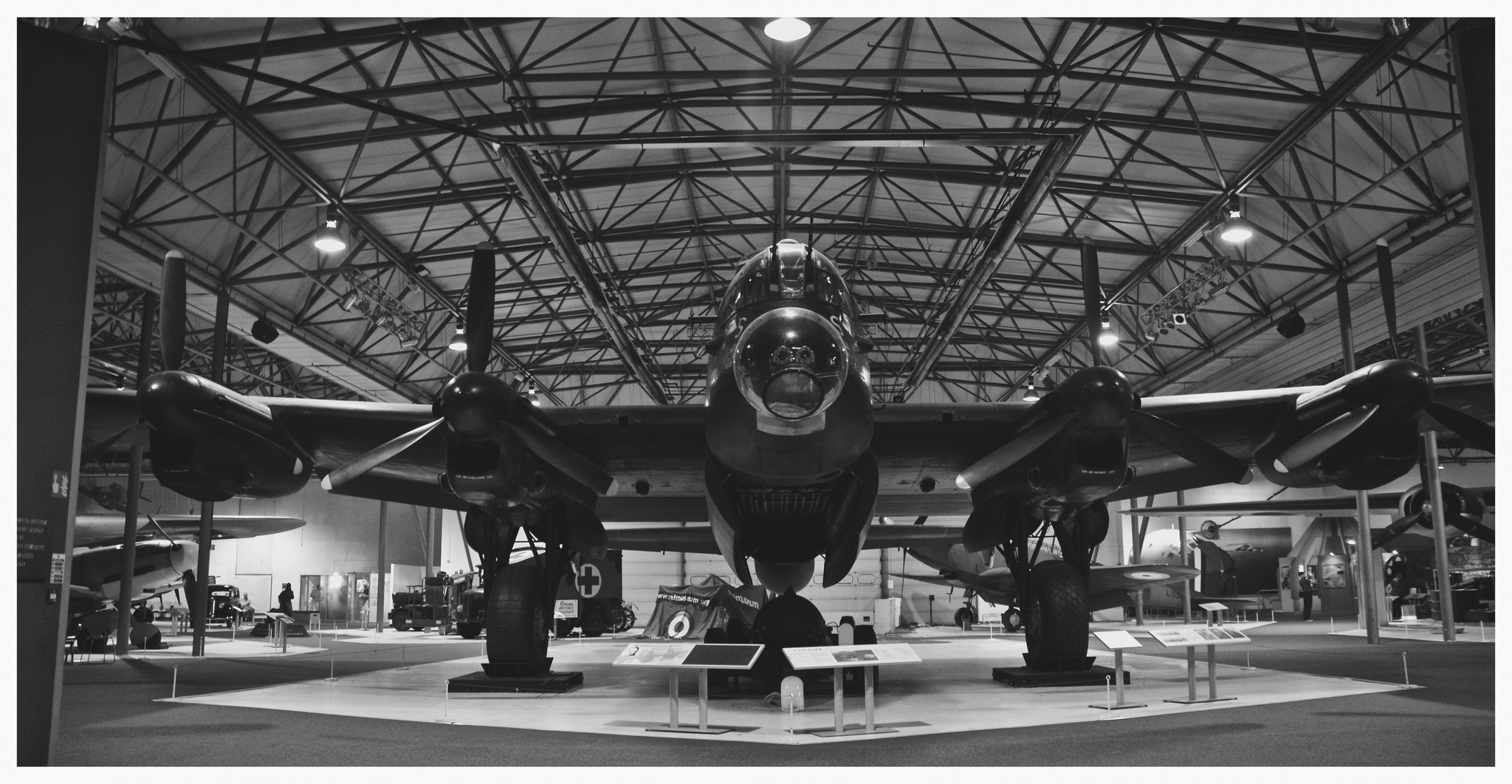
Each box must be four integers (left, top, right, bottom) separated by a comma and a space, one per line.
63, 205, 1496, 698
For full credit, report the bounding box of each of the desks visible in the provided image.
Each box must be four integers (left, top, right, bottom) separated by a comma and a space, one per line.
268, 610, 321, 631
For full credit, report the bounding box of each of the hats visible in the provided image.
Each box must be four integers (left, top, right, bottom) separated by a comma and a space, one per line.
1299, 572, 1305, 576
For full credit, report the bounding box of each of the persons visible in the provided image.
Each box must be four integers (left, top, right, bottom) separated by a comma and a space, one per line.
359, 581, 369, 620
240, 593, 251, 605
278, 583, 294, 611
1300, 573, 1315, 622
310, 584, 323, 611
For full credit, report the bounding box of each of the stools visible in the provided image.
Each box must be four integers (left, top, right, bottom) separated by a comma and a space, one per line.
80, 636, 116, 664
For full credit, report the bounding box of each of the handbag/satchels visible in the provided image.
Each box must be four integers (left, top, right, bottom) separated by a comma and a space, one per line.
1299, 591, 1305, 597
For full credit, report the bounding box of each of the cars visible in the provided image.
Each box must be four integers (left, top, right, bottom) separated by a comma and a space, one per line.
208, 585, 243, 628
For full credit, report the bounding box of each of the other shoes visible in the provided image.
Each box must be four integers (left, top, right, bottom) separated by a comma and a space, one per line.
1306, 620, 1314, 622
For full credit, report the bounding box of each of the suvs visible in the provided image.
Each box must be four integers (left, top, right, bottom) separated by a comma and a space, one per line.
455, 563, 487, 638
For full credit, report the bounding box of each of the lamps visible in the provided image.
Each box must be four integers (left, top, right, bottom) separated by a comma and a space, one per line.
1181, 221, 1213, 247
1045, 353, 1063, 367
693, 343, 706, 359
314, 204, 345, 251
1145, 271, 1230, 342
107, 17, 187, 80
1022, 375, 1040, 401
1100, 311, 1119, 344
526, 380, 540, 406
1382, 17, 1411, 42
1306, 17, 1340, 32
449, 316, 467, 351
764, 17, 811, 42
513, 371, 524, 382
338, 290, 358, 312
861, 296, 870, 313
358, 296, 419, 347
867, 249, 879, 270
415, 265, 431, 277
1221, 194, 1252, 240
